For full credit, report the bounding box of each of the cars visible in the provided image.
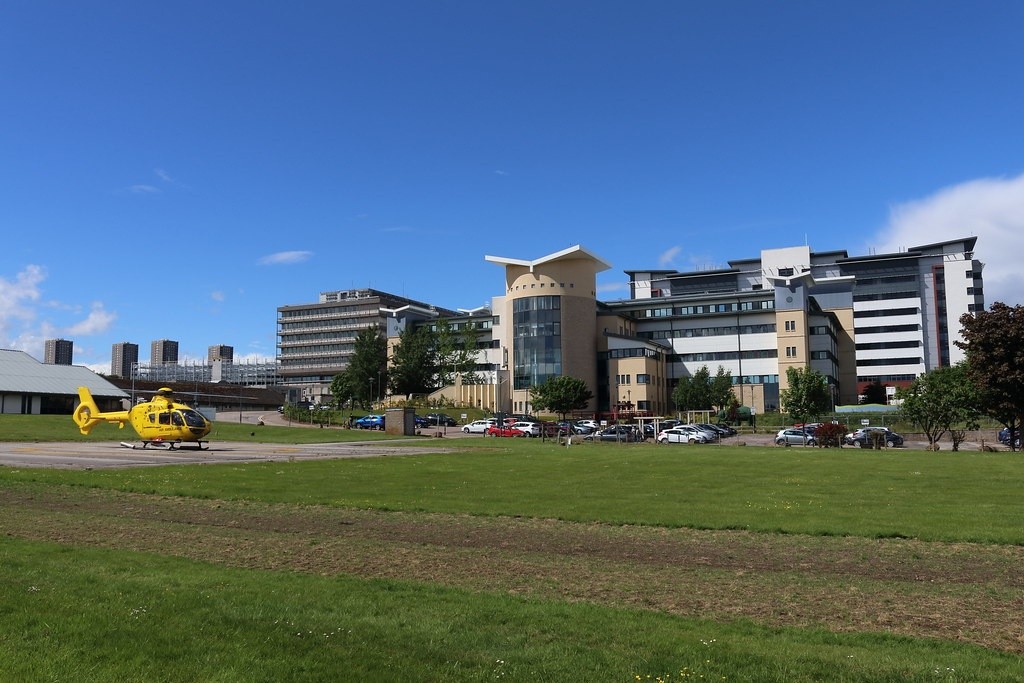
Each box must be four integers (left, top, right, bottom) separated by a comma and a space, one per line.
571, 422, 591, 435
488, 418, 522, 427
998, 423, 1019, 448
541, 422, 575, 436
775, 423, 823, 446
489, 425, 524, 437
657, 429, 705, 444
278, 402, 330, 414
584, 420, 681, 442
414, 418, 431, 428
672, 423, 736, 442
848, 431, 903, 447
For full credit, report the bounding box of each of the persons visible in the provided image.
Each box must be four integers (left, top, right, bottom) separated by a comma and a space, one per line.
349, 413, 354, 428
174, 411, 194, 425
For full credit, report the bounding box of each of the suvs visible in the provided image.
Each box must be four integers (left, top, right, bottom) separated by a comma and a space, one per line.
853, 428, 873, 438
510, 422, 540, 437
425, 413, 457, 427
845, 427, 888, 439
577, 420, 599, 430
462, 420, 494, 435
355, 415, 385, 430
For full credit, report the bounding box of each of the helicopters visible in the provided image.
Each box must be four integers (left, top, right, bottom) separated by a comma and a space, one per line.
73, 387, 259, 451
